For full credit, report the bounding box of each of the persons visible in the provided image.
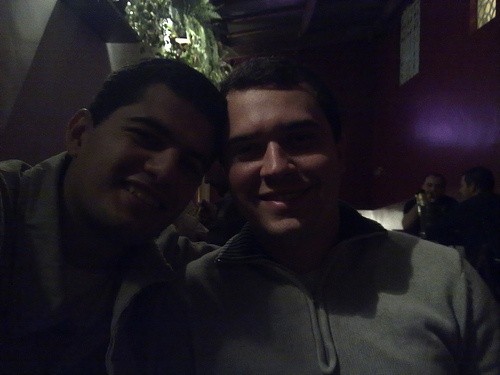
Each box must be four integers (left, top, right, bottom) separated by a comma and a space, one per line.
196, 166, 242, 242
0, 59, 230, 374
164, 58, 500, 374
428, 167, 500, 274
403, 174, 456, 230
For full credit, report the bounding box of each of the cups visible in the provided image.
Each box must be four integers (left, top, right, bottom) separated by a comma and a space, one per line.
122, 0, 230, 88
